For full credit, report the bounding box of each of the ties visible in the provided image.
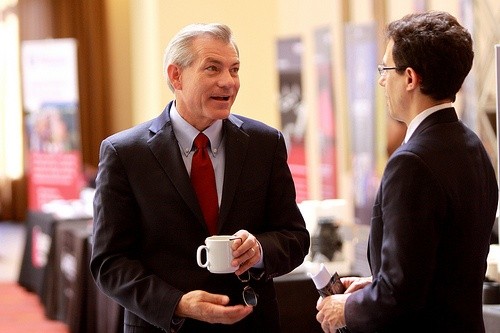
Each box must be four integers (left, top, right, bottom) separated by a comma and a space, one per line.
189, 133, 219, 236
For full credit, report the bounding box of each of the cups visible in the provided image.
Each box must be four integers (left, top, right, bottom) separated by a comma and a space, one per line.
197, 235, 239, 273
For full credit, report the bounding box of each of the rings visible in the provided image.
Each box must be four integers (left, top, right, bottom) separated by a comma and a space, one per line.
252, 248, 256, 254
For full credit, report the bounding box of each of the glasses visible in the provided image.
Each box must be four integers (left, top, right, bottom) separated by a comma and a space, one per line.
377, 64, 408, 75
230, 237, 259, 308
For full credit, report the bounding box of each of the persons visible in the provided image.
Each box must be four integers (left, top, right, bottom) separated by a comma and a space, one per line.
315, 9, 499, 333
90, 23, 309, 333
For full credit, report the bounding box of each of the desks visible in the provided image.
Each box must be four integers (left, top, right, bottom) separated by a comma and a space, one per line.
17, 210, 325, 333
